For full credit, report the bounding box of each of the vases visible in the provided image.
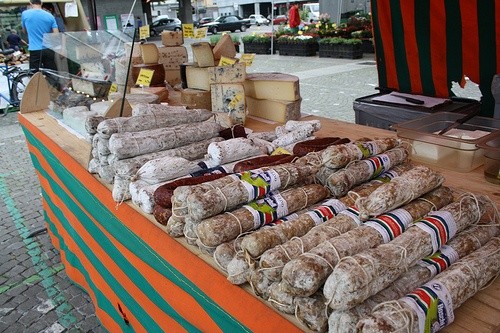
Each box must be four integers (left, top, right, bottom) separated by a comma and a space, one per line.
211, 22, 374, 59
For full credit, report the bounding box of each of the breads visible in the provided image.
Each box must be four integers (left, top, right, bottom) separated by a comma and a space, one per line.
125, 31, 302, 123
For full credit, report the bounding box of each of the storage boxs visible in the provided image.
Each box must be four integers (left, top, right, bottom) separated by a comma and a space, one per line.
352, 89, 480, 132
393, 108, 500, 184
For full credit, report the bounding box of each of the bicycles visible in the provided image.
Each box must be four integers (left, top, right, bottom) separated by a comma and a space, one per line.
0, 58, 35, 117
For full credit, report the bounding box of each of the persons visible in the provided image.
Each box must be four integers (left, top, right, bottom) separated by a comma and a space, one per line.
136, 17, 147, 44
41, 2, 65, 33
7, 29, 29, 51
288, 1, 309, 35
127, 20, 134, 28
21, 0, 61, 92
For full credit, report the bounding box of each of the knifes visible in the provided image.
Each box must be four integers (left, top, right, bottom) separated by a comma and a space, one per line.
392, 94, 424, 104
438, 110, 480, 134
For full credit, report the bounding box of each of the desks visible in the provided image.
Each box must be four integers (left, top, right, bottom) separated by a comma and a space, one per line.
18, 111, 500, 333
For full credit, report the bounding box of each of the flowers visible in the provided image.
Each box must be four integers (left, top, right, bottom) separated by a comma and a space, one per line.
221, 12, 372, 40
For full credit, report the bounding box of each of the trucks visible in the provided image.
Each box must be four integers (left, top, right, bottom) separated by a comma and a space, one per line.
300, 3, 320, 25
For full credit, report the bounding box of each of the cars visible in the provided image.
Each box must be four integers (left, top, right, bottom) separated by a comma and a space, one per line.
198, 15, 252, 35
149, 18, 182, 38
243, 14, 270, 26
273, 15, 287, 25
267, 15, 272, 22
194, 17, 215, 26
